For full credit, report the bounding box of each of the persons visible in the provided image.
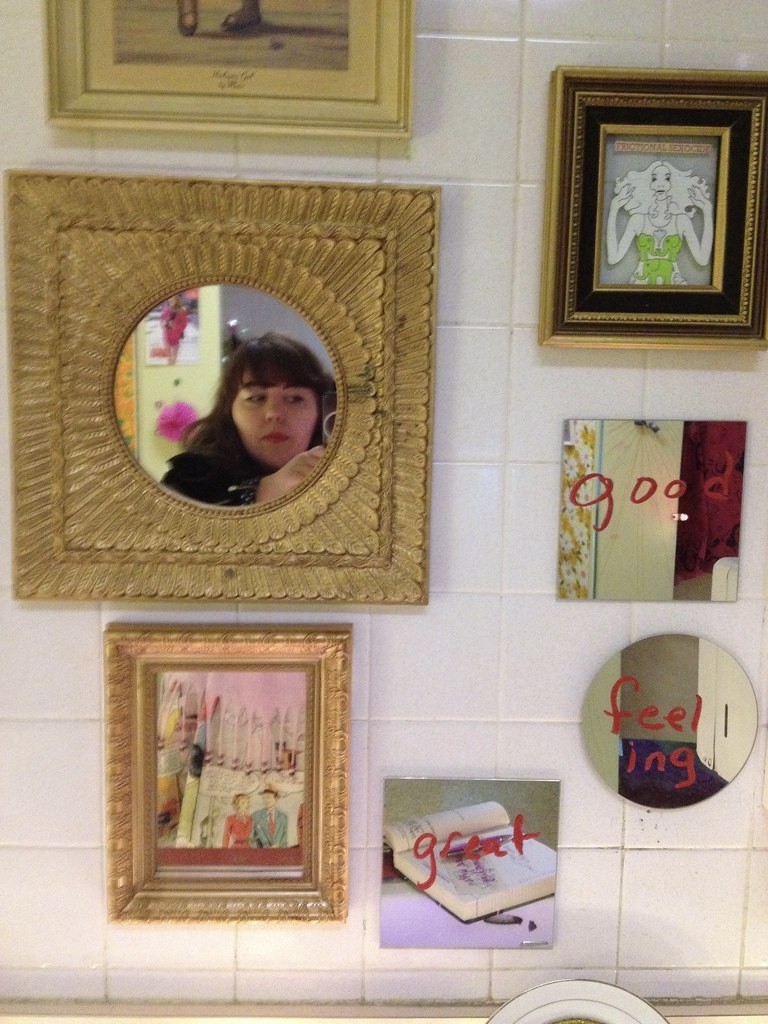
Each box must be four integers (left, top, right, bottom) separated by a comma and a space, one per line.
161, 332, 337, 508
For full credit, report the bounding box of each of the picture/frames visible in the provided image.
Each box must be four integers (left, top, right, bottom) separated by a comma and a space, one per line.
541, 60, 768, 350
104, 619, 350, 923
45, 0, 416, 139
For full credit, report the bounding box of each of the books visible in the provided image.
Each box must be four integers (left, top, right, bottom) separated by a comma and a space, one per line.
382, 800, 558, 923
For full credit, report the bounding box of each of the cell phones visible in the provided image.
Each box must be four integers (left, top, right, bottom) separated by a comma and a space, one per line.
323, 392, 338, 449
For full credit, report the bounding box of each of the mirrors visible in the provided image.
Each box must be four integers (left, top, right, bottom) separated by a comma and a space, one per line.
11, 170, 441, 609
